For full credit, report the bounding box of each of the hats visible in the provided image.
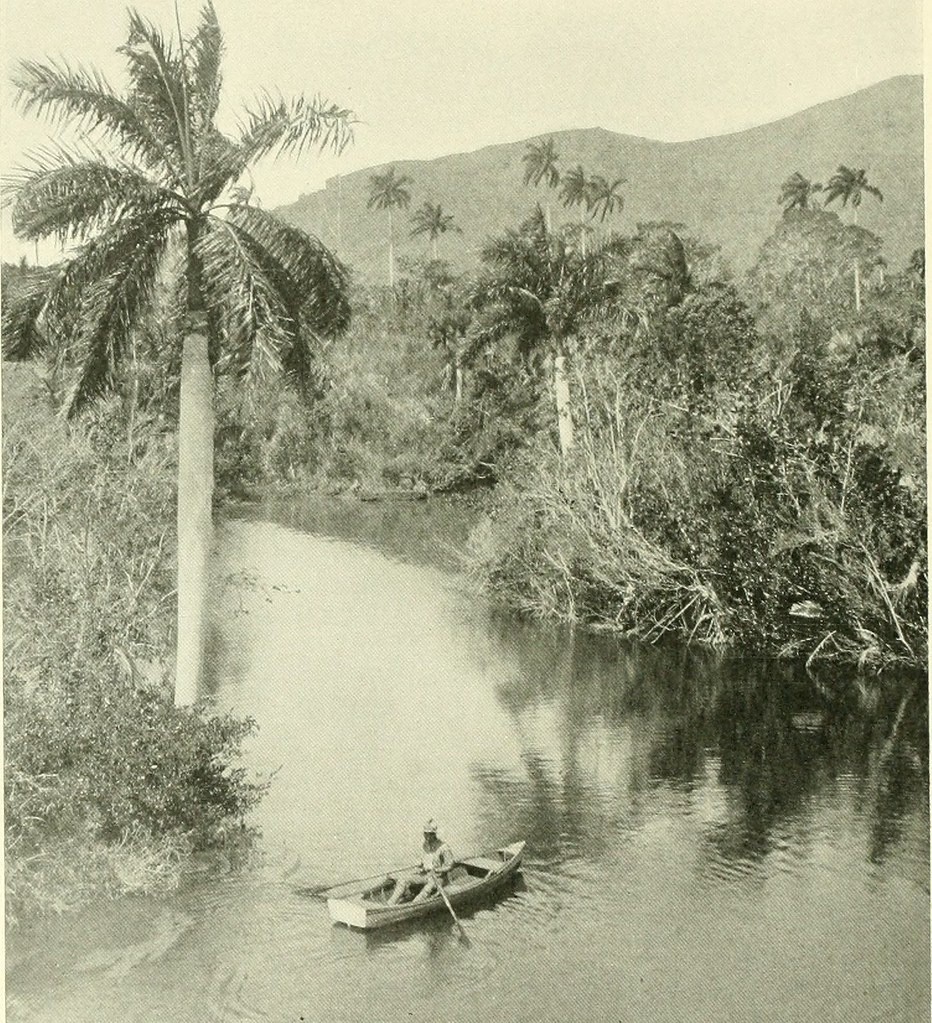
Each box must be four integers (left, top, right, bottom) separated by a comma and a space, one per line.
421, 818, 439, 834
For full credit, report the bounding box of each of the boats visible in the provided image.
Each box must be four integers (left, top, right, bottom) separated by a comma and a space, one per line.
327, 840, 527, 929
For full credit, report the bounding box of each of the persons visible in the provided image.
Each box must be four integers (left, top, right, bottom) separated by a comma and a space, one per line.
385, 818, 459, 905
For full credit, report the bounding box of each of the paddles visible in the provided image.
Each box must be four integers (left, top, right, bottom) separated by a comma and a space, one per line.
424, 860, 475, 950
294, 858, 425, 899
